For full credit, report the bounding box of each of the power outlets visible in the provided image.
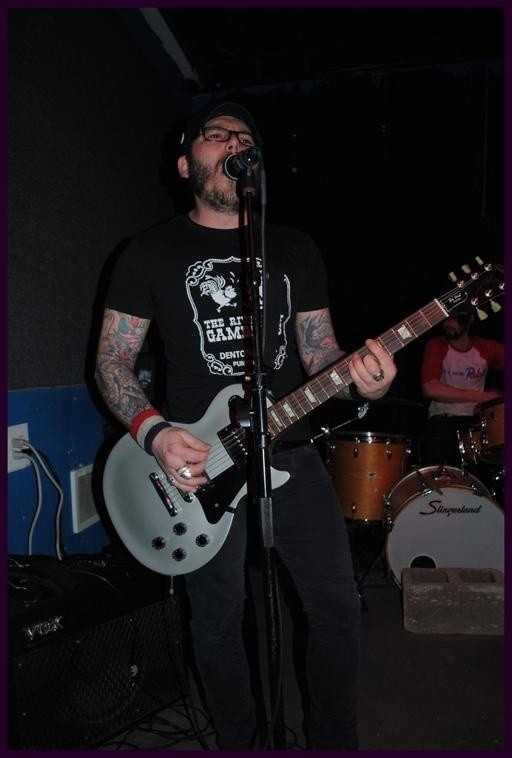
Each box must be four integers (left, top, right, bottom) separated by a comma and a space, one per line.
8, 424, 30, 477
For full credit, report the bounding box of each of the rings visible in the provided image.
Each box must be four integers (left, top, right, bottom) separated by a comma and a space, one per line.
373, 371, 384, 381
167, 477, 176, 486
176, 465, 192, 480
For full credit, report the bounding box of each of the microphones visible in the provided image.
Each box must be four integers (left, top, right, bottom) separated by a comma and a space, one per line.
223, 145, 263, 181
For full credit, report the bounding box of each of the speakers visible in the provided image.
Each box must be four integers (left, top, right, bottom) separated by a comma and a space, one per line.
7, 545, 191, 751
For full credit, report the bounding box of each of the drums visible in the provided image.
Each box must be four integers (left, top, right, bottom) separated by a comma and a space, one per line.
470, 396, 506, 456
461, 429, 483, 462
382, 465, 507, 588
324, 432, 411, 523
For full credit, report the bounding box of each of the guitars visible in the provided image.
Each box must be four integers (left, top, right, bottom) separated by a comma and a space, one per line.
101, 253, 504, 577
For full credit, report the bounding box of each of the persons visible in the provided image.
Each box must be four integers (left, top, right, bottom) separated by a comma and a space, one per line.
420, 303, 505, 470
94, 101, 397, 750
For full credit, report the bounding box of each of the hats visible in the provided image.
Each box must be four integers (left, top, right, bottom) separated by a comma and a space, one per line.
175, 93, 262, 157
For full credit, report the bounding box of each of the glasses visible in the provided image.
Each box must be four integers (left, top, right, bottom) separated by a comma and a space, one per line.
197, 126, 259, 148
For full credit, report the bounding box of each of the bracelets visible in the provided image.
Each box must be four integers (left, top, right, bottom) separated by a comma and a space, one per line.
129, 409, 171, 456
